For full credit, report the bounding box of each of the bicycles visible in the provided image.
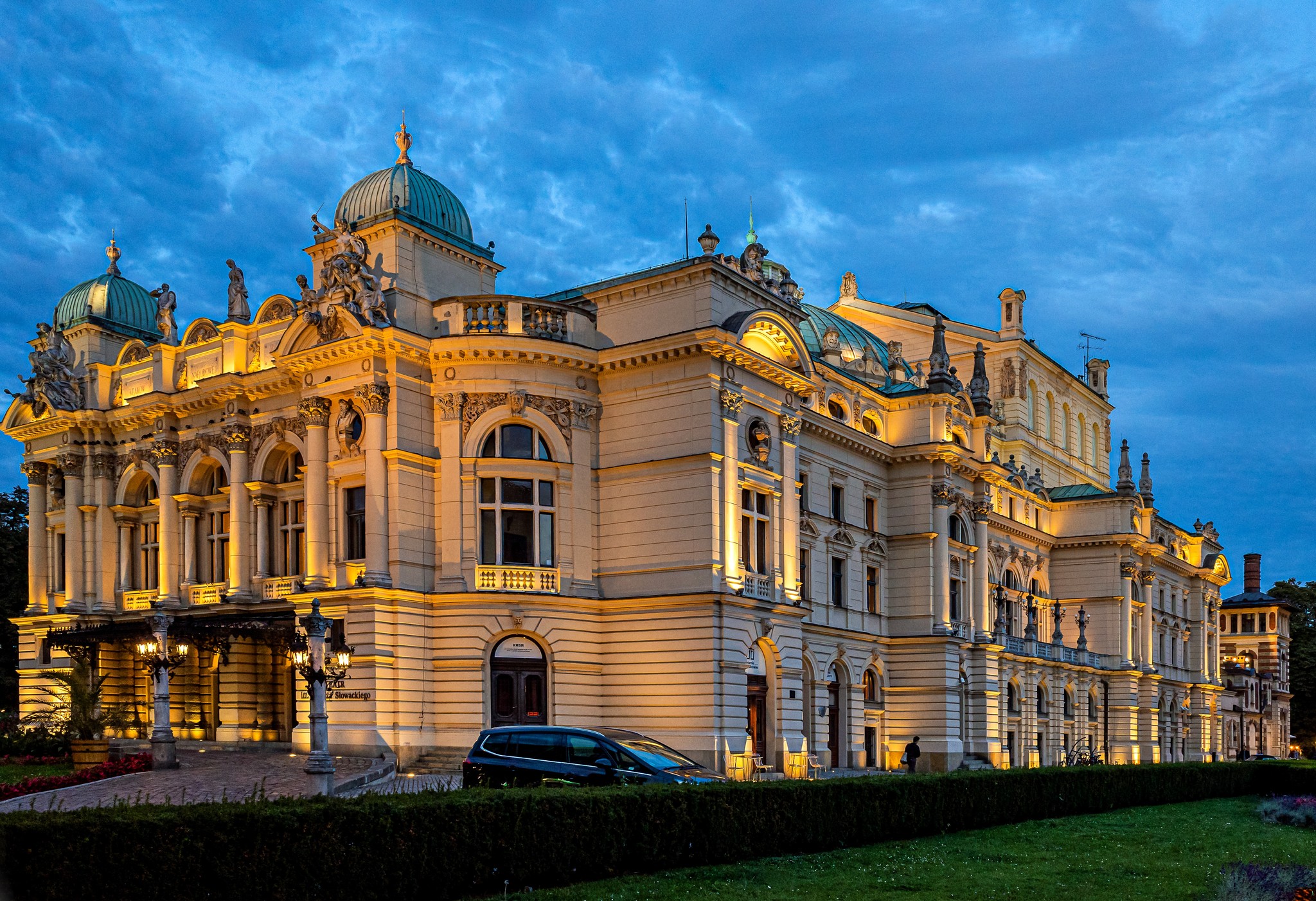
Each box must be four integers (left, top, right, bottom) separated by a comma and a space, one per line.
1058, 750, 1104, 767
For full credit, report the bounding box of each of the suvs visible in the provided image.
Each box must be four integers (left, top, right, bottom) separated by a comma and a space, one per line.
462, 725, 733, 793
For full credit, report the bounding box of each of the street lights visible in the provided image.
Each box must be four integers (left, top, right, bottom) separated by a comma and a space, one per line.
1022, 591, 1039, 641
992, 580, 1008, 633
1075, 605, 1090, 650
135, 601, 189, 767
288, 598, 355, 796
1051, 597, 1066, 645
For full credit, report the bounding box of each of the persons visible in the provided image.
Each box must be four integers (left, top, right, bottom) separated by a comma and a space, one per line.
1238, 745, 1250, 761
1256, 750, 1264, 755
904, 736, 920, 773
